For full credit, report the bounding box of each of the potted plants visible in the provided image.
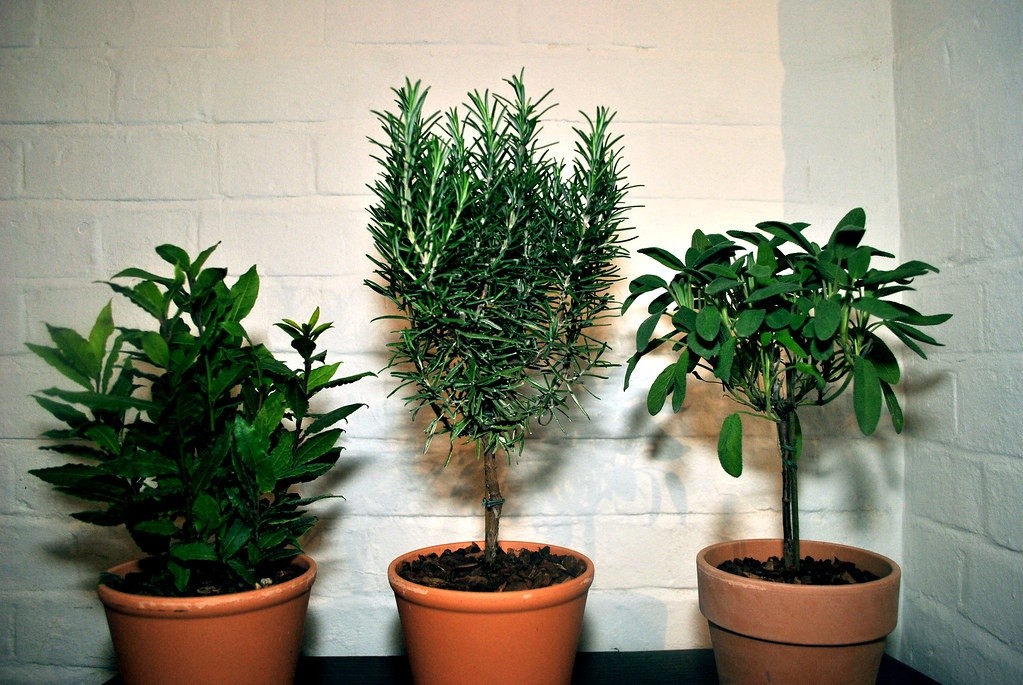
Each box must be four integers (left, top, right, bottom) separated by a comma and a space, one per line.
366, 66, 644, 685
620, 207, 954, 685
23, 241, 375, 685
